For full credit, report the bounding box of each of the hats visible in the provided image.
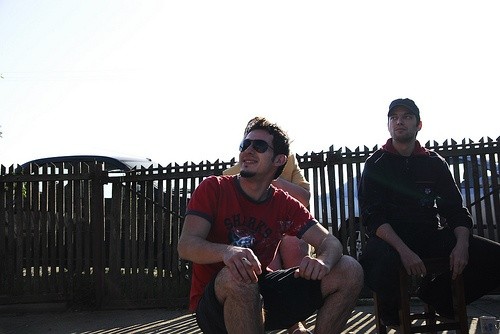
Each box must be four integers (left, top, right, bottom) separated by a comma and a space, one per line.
387, 98, 420, 118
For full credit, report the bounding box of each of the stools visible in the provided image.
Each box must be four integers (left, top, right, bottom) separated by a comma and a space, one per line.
370, 254, 468, 334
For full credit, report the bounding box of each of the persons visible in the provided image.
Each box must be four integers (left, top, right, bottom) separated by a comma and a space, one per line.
359, 99, 500, 329
222, 116, 316, 334
177, 118, 364, 333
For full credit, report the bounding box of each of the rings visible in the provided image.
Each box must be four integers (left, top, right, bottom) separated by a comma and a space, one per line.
241, 258, 247, 261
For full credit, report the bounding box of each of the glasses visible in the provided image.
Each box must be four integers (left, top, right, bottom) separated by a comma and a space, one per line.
239, 139, 280, 156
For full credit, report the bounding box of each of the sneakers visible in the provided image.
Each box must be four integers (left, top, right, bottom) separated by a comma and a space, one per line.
416, 282, 454, 319
380, 300, 401, 327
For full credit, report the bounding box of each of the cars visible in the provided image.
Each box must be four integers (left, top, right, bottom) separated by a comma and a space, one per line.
312, 154, 500, 264
0, 154, 203, 269
